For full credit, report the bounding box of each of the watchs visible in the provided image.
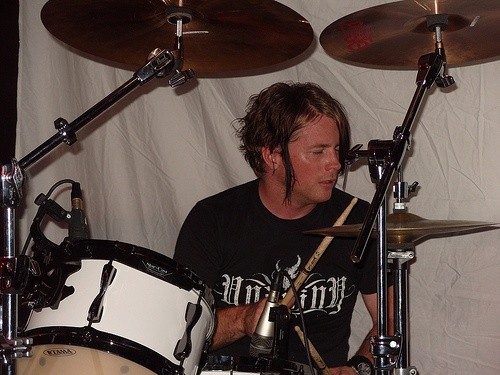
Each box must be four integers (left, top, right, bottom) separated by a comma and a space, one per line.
347, 355, 375, 374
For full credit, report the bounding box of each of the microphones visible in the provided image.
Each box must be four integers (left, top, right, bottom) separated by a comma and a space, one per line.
68, 182, 89, 242
249, 272, 284, 358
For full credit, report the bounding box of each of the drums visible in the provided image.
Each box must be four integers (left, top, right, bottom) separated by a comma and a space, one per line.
10, 238, 218, 375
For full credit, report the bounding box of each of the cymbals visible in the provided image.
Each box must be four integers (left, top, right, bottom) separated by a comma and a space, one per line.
320, 0, 499, 69
306, 212, 500, 246
40, 0, 316, 78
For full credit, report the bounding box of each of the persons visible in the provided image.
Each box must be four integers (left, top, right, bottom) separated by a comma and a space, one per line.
171, 82, 394, 375
